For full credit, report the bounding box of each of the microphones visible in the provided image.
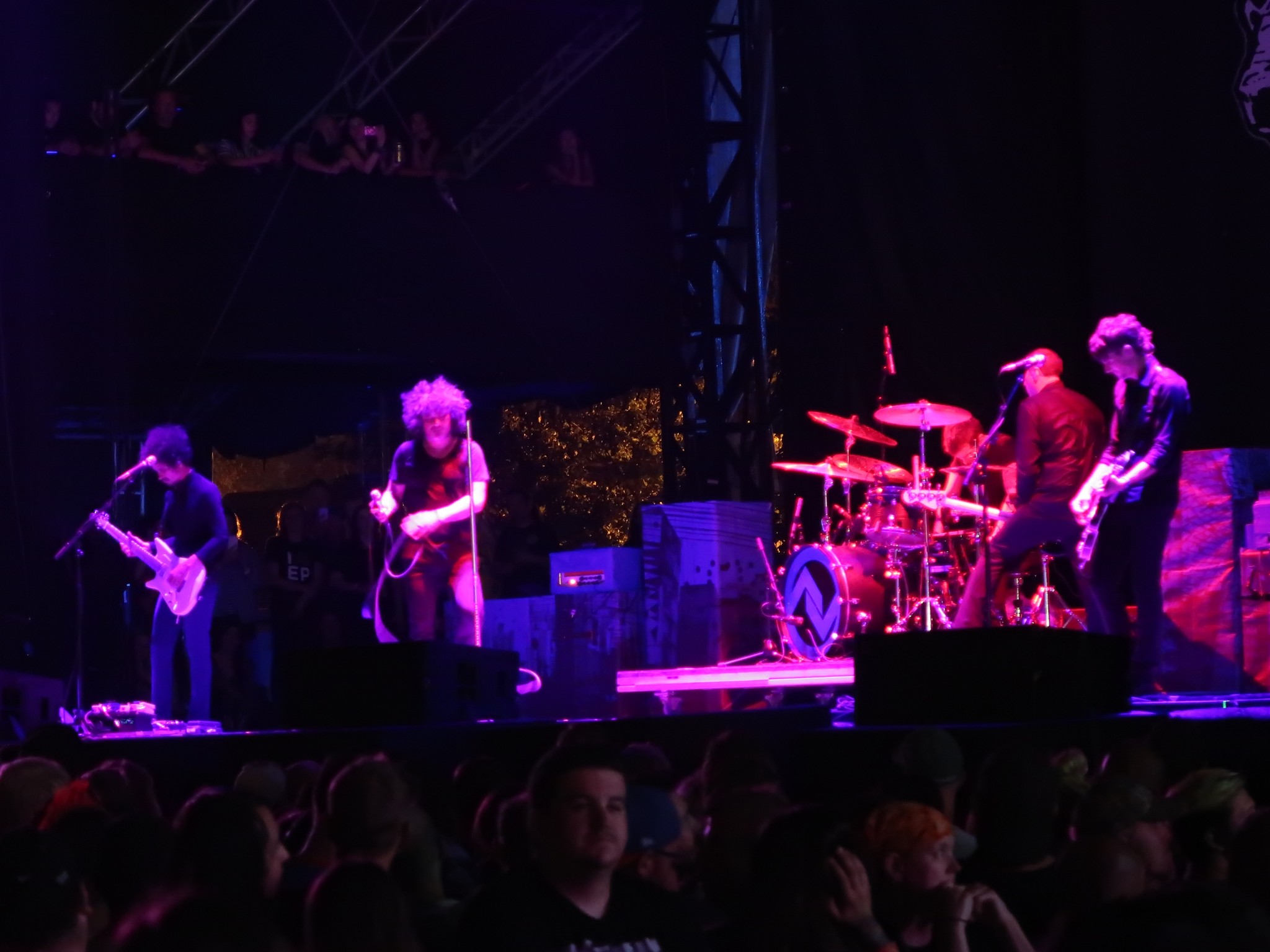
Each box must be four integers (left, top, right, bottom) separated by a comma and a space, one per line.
769, 614, 804, 627
369, 489, 392, 532
999, 355, 1046, 373
115, 455, 156, 482
884, 325, 897, 373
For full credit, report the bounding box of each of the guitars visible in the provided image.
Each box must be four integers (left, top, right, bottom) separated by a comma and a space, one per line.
1072, 448, 1132, 569
900, 484, 1023, 542
91, 507, 205, 615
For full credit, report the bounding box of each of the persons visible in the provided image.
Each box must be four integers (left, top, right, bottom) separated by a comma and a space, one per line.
120, 426, 238, 720
367, 377, 489, 645
952, 349, 1107, 627
0, 736, 1269, 952
1068, 313, 1192, 694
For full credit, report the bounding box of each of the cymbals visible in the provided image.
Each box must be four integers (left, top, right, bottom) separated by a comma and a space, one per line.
874, 400, 971, 428
824, 454, 914, 482
938, 466, 1013, 473
770, 460, 873, 482
805, 409, 898, 448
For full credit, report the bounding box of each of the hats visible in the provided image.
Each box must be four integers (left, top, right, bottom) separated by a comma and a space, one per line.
998, 349, 1064, 375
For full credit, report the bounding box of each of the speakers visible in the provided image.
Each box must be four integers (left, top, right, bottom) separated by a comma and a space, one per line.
0, 672, 69, 744
272, 642, 518, 725
853, 629, 1130, 721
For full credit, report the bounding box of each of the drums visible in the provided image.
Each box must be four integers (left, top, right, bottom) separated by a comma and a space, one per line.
927, 528, 987, 578
783, 545, 902, 664
864, 483, 936, 549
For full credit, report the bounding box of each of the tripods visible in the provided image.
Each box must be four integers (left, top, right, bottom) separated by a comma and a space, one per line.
1018, 553, 1089, 629
718, 537, 808, 666
880, 425, 952, 631
